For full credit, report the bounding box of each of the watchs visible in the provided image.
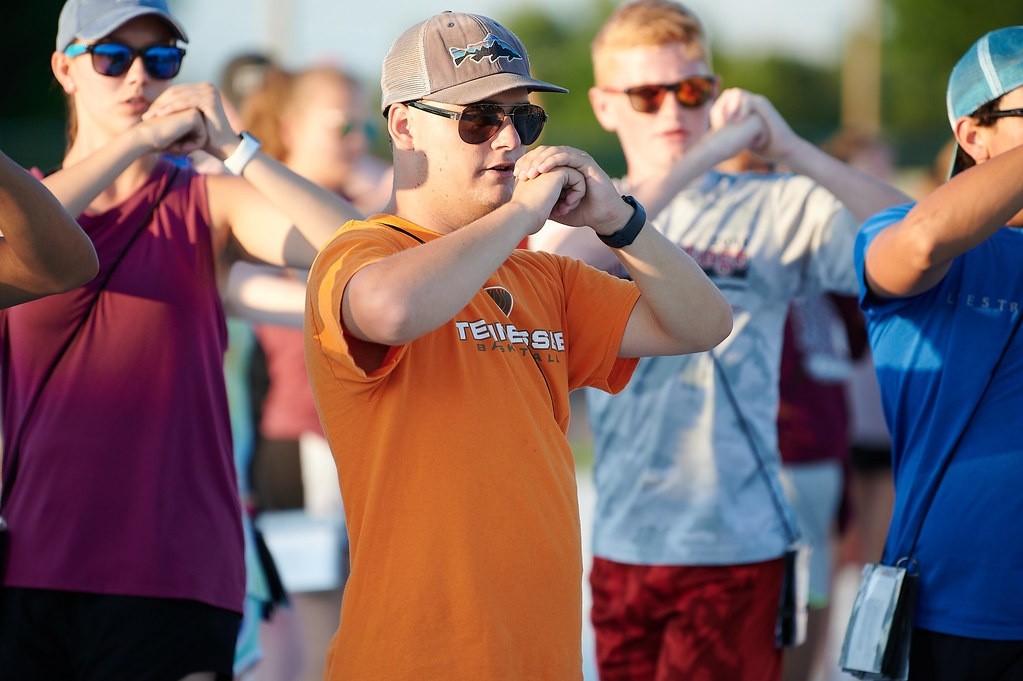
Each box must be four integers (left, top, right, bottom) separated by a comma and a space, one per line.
222, 130, 262, 176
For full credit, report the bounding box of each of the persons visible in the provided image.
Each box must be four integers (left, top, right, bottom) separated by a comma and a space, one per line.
0, 150, 99, 314
219, 47, 904, 681
0, 0, 365, 681
302, 9, 734, 681
538, 0, 917, 681
852, 26, 1023, 680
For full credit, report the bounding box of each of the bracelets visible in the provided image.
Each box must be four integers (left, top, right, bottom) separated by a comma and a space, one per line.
596, 194, 646, 248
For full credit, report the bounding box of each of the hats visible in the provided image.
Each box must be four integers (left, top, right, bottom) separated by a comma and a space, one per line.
946, 26, 1023, 188
56, 0, 189, 52
380, 11, 569, 118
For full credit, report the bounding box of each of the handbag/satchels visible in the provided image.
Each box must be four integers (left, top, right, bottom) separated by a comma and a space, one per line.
781, 544, 807, 646
839, 561, 912, 681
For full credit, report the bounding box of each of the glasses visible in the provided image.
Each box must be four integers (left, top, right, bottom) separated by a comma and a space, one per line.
402, 101, 548, 144
597, 76, 719, 114
63, 41, 186, 80
